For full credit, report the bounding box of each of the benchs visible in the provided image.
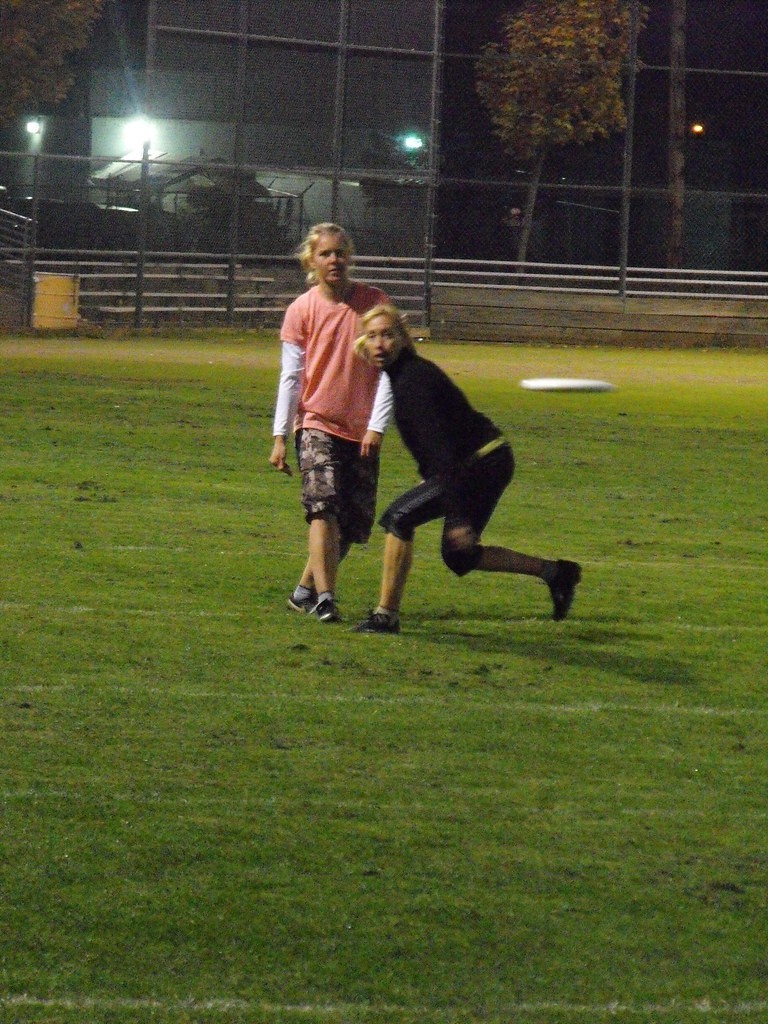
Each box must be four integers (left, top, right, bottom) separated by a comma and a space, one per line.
343, 256, 768, 315
5, 260, 299, 321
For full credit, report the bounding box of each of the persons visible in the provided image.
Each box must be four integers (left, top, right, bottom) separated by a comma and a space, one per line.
343, 305, 581, 633
269, 223, 396, 622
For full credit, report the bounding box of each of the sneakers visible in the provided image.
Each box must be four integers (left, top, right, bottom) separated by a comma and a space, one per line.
547, 559, 581, 620
288, 595, 316, 614
353, 609, 400, 633
315, 598, 337, 622
